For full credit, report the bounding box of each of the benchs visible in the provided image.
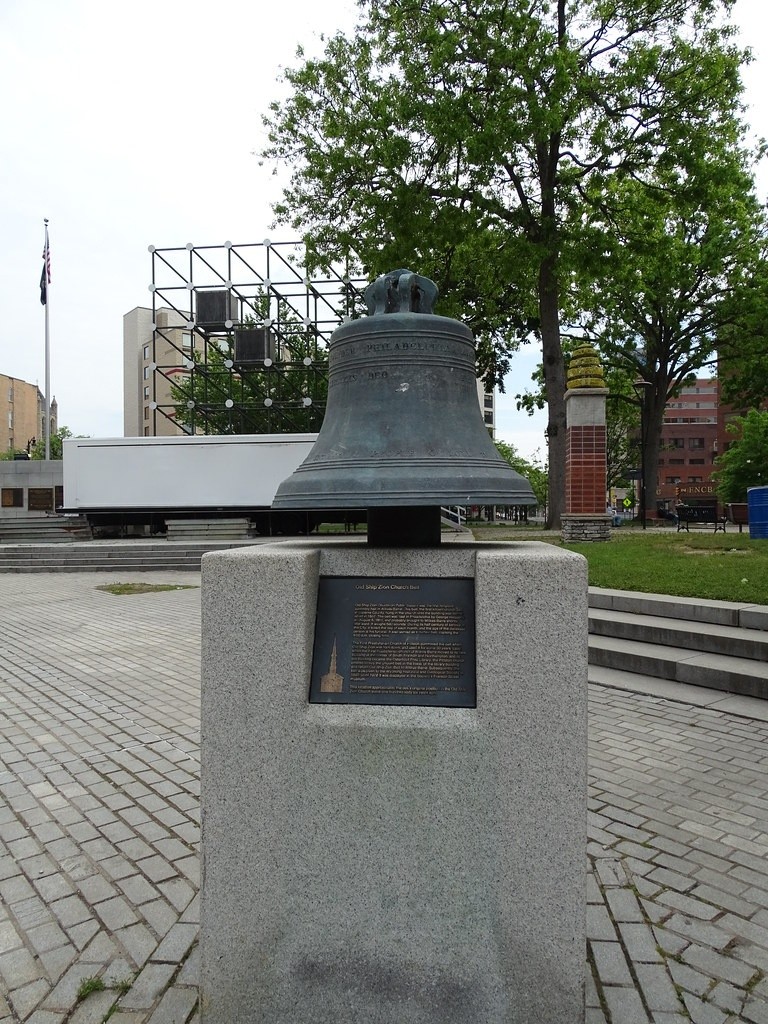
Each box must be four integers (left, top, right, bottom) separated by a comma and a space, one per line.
726, 503, 749, 533
645, 509, 668, 528
675, 505, 726, 533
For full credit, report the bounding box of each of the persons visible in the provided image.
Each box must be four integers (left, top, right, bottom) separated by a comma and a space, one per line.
607, 502, 621, 526
658, 506, 677, 526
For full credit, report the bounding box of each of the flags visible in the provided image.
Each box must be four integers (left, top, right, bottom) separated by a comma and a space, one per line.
40, 230, 51, 306
